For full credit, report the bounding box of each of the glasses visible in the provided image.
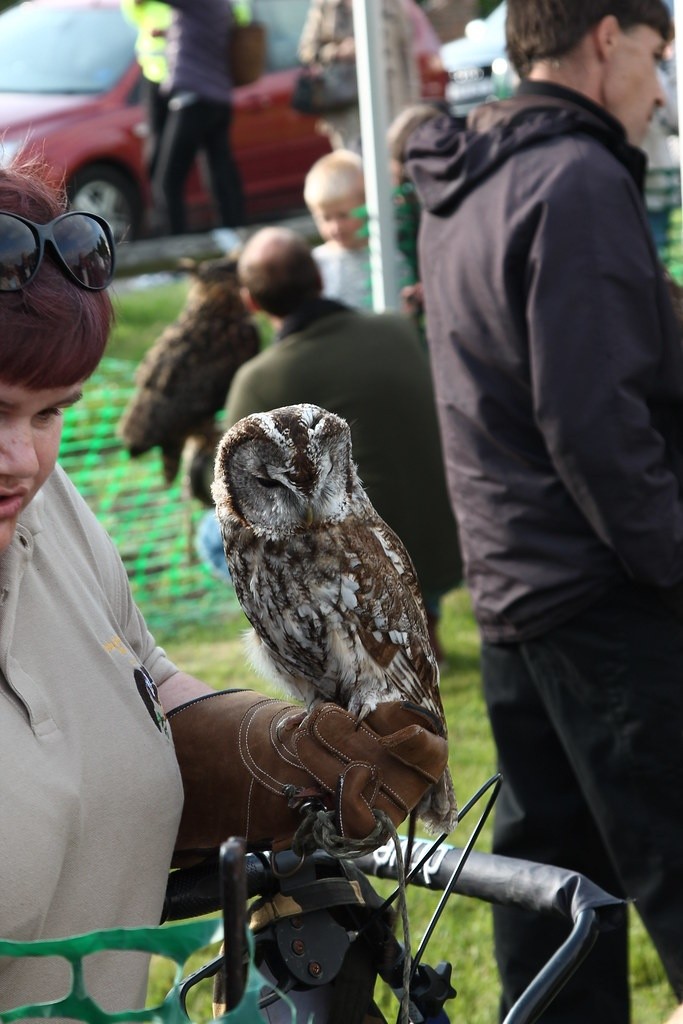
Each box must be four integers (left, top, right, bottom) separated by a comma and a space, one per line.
0, 211, 116, 291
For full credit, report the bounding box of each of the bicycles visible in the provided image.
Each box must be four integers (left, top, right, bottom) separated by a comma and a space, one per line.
155, 771, 629, 1024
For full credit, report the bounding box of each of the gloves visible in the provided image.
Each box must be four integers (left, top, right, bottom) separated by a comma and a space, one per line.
173, 691, 450, 848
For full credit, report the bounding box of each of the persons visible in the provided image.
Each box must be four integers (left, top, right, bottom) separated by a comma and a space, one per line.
408, 0, 683, 1023
120, 0, 683, 656
0, 170, 448, 1024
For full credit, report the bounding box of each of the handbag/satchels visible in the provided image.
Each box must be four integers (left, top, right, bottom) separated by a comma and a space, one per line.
231, 22, 264, 85
292, 55, 358, 113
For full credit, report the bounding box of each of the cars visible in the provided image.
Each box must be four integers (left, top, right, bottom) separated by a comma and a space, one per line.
0, 1, 451, 241
435, 2, 677, 122
